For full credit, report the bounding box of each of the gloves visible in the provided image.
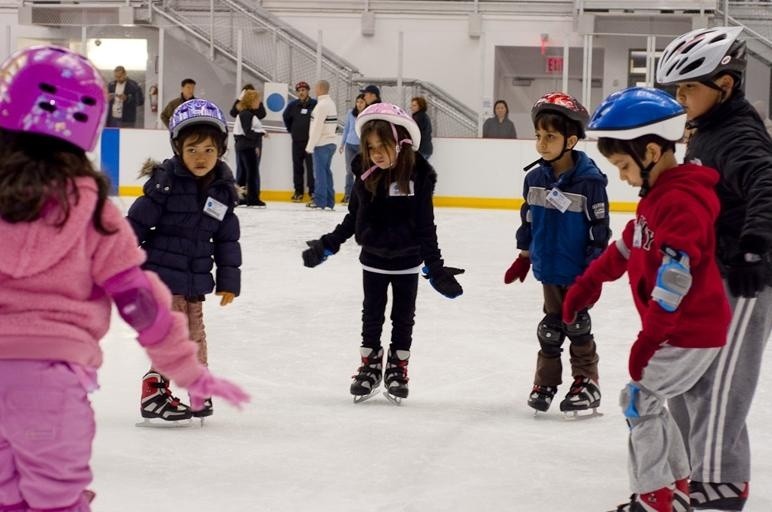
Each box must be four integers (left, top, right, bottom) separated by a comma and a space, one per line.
563, 277, 601, 325
728, 236, 772, 298
629, 331, 668, 381
215, 292, 235, 306
505, 253, 530, 283
188, 370, 251, 412
303, 233, 339, 267
420, 259, 464, 298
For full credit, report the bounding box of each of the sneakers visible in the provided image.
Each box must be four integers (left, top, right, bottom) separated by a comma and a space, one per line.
141, 373, 194, 420
350, 348, 382, 395
607, 477, 748, 512
237, 192, 266, 208
559, 378, 600, 411
384, 344, 408, 397
290, 194, 349, 208
188, 392, 213, 417
527, 383, 557, 411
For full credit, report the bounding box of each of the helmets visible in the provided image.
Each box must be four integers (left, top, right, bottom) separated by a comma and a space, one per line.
169, 99, 227, 139
533, 26, 745, 142
355, 103, 421, 151
0, 46, 108, 151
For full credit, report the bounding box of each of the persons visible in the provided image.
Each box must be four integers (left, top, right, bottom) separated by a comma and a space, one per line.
651, 26, 769, 511
301, 103, 465, 399
481, 99, 519, 138
563, 85, 735, 512
126, 99, 243, 419
339, 95, 369, 204
230, 83, 266, 190
0, 45, 249, 510
160, 78, 201, 133
358, 85, 383, 110
408, 97, 433, 162
233, 90, 267, 206
104, 65, 144, 128
282, 81, 318, 201
305, 80, 337, 208
506, 88, 612, 411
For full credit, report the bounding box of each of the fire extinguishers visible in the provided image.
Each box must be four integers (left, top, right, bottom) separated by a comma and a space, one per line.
149, 83, 157, 112
